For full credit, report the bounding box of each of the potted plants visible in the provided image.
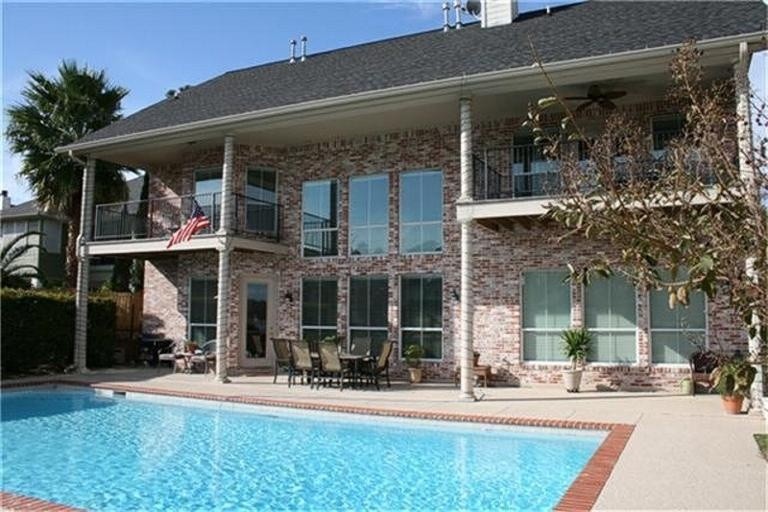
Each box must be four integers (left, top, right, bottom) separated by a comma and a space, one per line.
403, 344, 425, 384
474, 352, 480, 366
185, 340, 197, 351
559, 327, 596, 393
715, 358, 758, 414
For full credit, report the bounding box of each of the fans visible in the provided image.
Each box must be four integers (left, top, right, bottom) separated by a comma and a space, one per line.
564, 85, 626, 111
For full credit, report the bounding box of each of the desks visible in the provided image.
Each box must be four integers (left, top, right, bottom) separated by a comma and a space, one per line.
455, 365, 491, 388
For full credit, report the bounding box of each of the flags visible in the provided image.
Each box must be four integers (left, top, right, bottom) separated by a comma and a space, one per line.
165, 196, 212, 250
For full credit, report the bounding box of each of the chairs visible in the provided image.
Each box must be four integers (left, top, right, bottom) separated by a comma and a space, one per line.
269, 336, 398, 391
189, 339, 216, 376
689, 350, 719, 396
155, 337, 188, 375
